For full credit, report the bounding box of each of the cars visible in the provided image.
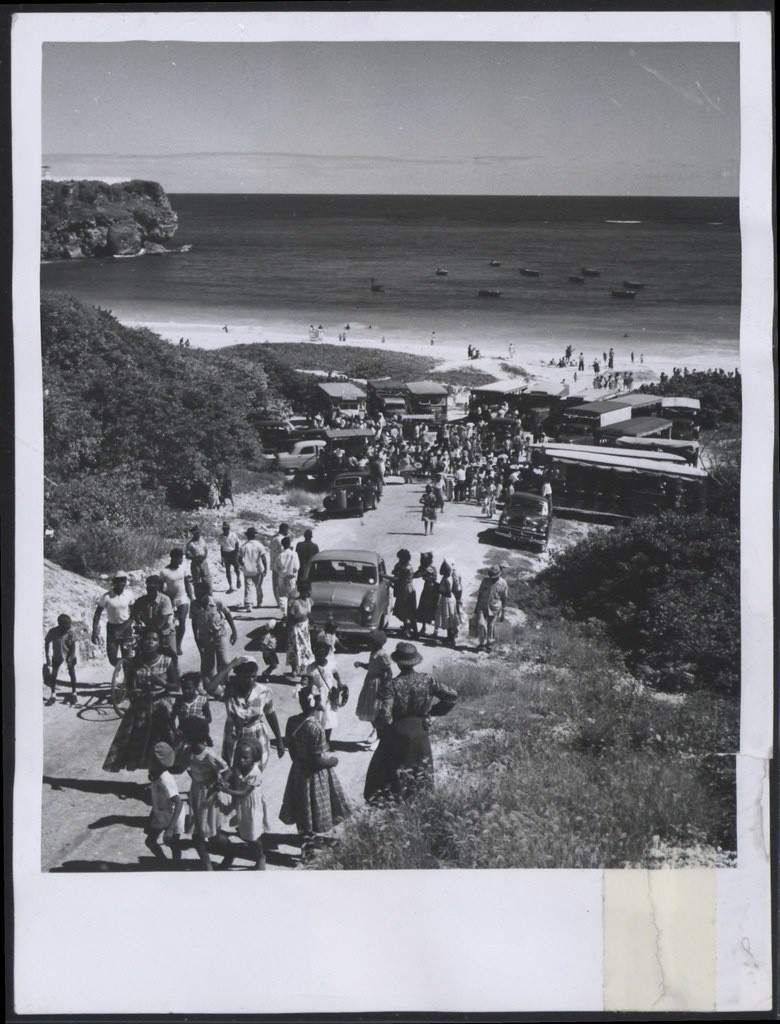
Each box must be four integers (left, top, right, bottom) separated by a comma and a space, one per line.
497, 492, 556, 553
325, 473, 382, 515
252, 421, 293, 448
306, 550, 391, 642
274, 440, 328, 475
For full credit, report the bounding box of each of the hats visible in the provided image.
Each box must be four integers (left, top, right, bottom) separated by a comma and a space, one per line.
265, 619, 277, 631
221, 521, 230, 527
244, 527, 258, 534
391, 642, 422, 666
237, 737, 263, 762
152, 741, 176, 768
487, 565, 501, 579
114, 570, 128, 578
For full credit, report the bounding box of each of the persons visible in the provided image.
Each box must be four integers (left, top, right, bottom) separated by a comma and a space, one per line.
41, 324, 645, 871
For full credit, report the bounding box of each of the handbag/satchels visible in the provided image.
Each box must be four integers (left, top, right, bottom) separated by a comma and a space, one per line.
492, 617, 512, 641
468, 613, 482, 637
315, 664, 340, 711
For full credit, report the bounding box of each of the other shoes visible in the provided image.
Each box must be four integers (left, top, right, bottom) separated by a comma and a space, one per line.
69, 694, 77, 704
45, 696, 56, 706
237, 580, 241, 589
226, 588, 234, 594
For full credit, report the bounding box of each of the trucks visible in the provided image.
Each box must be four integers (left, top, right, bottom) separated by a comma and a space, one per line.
303, 428, 376, 456
369, 379, 411, 419
317, 382, 368, 423
402, 380, 451, 424
468, 378, 711, 527
